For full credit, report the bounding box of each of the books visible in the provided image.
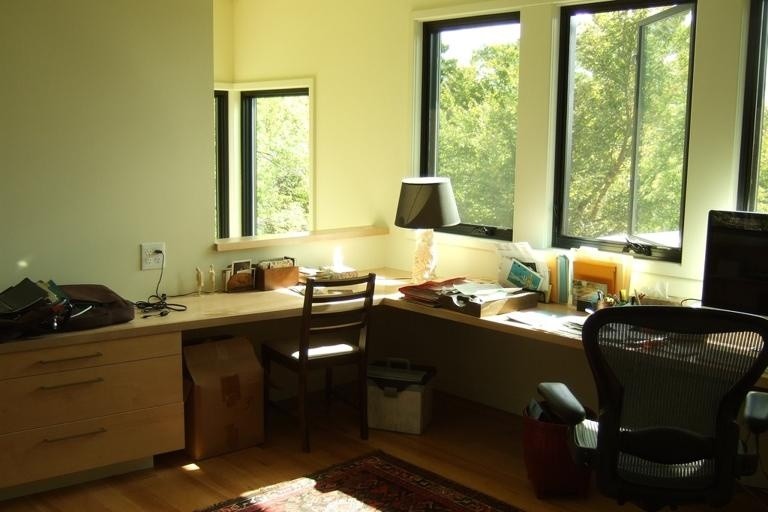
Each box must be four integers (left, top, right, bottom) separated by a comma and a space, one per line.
398, 241, 634, 319
1, 276, 69, 316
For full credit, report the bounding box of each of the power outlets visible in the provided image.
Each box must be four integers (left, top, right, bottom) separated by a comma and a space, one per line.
141, 243, 166, 270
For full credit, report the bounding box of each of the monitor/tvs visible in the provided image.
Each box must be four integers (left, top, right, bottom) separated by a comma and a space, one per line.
700, 209, 768, 316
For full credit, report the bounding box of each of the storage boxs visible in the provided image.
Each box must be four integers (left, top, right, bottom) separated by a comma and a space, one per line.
366, 380, 434, 436
183, 337, 265, 461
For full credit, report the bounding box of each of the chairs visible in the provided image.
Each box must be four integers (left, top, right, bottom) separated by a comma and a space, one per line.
262, 272, 376, 453
538, 306, 768, 512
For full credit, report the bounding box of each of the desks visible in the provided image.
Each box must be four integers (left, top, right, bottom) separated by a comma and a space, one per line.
0, 268, 768, 502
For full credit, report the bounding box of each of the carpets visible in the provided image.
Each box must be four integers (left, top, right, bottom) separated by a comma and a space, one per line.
201, 449, 527, 512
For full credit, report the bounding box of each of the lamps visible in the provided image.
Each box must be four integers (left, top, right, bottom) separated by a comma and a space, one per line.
394, 177, 461, 285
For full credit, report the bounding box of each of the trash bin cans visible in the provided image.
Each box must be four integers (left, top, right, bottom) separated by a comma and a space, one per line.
523, 400, 598, 500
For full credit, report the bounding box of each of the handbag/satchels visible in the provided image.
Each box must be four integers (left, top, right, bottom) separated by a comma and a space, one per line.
0, 277, 69, 343
57, 283, 135, 334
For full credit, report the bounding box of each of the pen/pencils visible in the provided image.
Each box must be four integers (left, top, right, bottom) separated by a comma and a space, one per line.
598, 287, 645, 306
